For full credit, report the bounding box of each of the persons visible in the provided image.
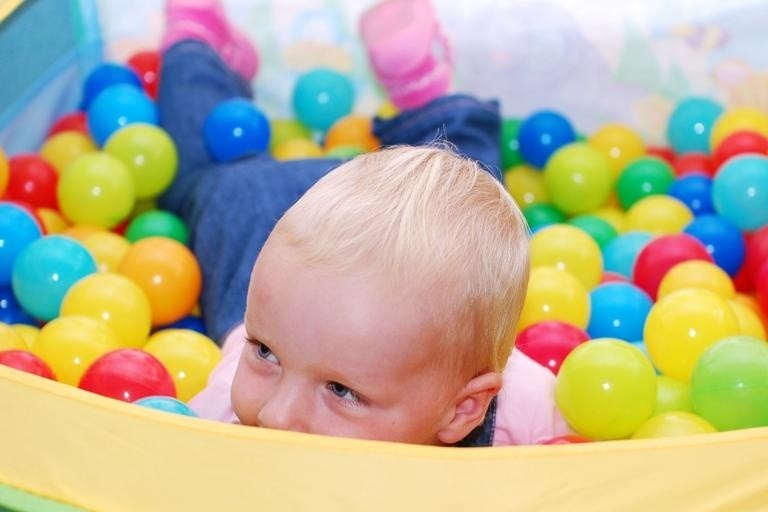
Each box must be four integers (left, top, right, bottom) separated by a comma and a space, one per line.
160, 0, 531, 443
